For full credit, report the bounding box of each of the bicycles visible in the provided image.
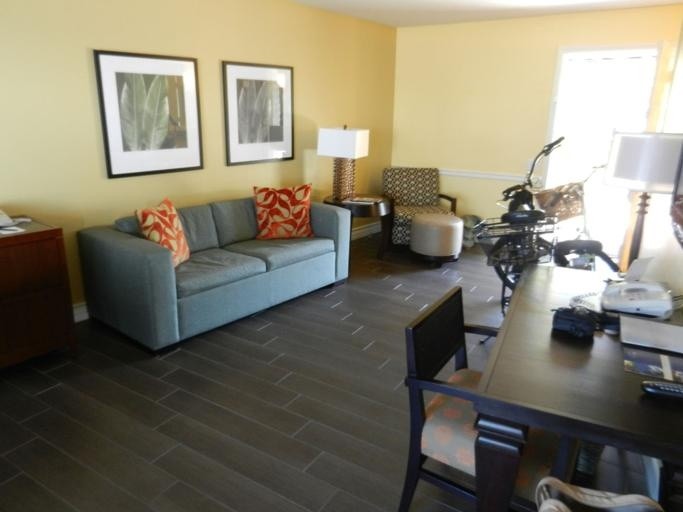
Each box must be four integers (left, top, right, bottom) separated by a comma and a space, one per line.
471, 135, 620, 316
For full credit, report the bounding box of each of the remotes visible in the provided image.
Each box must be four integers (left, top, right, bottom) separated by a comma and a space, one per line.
640, 380, 682, 398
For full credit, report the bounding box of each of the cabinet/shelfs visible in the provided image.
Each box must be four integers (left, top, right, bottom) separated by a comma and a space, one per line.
0, 214, 73, 375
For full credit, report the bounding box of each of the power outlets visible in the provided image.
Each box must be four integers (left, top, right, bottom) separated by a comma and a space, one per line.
74, 302, 90, 323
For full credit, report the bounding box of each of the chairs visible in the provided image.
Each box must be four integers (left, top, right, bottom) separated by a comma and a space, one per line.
398, 286, 583, 512
382, 167, 457, 252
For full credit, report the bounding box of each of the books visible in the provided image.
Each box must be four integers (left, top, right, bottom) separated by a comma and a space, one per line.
342, 196, 382, 206
619, 313, 682, 382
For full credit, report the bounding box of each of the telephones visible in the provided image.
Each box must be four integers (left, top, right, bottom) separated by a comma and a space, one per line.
569, 281, 673, 319
1, 210, 32, 226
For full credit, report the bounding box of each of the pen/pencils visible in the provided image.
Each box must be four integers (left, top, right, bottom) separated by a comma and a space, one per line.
1, 228, 18, 232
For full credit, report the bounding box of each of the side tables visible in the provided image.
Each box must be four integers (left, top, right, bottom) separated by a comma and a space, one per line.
321, 192, 390, 242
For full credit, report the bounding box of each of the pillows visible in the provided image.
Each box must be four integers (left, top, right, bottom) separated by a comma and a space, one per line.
252, 181, 313, 240
135, 199, 191, 268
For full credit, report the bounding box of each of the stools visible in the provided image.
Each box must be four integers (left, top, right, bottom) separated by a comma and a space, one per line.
411, 213, 464, 261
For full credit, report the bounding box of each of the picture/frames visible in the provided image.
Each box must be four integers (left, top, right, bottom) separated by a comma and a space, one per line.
92, 49, 205, 179
221, 60, 295, 167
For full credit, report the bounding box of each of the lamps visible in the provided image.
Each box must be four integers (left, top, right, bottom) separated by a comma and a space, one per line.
610, 133, 682, 271
317, 124, 369, 200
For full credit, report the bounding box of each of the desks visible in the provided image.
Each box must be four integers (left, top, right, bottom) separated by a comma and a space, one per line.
475, 263, 683, 512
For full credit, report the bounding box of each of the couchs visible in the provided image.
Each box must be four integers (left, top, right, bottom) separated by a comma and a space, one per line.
76, 197, 351, 351
